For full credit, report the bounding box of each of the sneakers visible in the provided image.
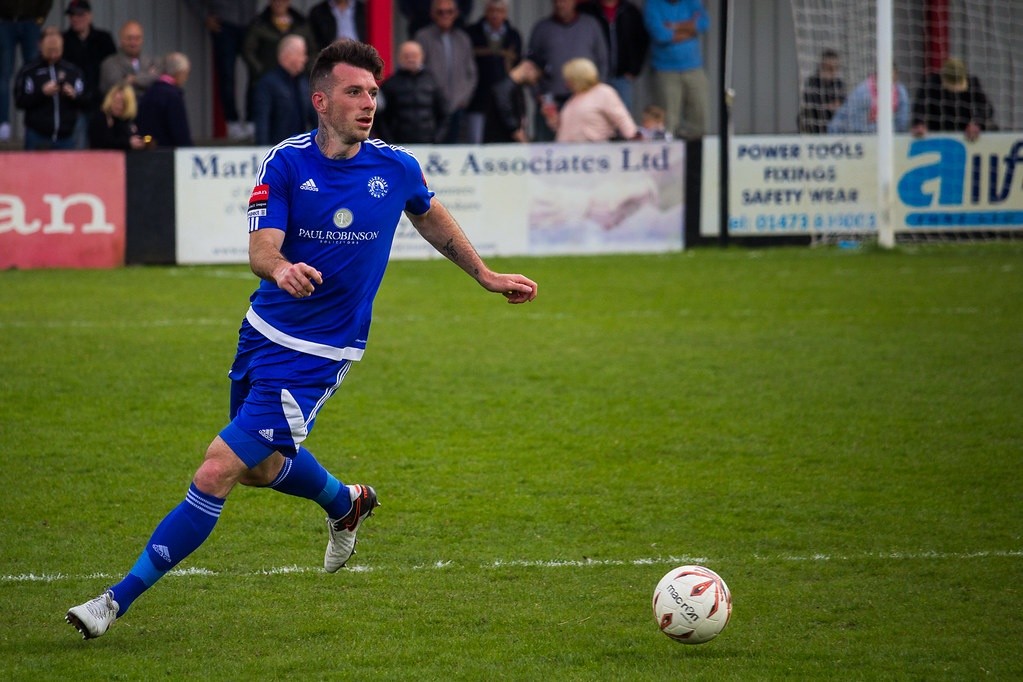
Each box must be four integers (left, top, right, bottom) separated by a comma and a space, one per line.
323, 485, 381, 574
64, 588, 119, 639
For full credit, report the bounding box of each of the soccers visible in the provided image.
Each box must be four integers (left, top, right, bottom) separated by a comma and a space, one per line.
652, 565, 733, 645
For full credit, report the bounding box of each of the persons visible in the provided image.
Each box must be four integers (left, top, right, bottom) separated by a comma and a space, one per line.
795, 48, 847, 134
0, 0, 667, 266
13, 26, 84, 150
556, 58, 640, 141
644, 0, 713, 136
912, 57, 999, 143
381, 40, 448, 143
67, 38, 537, 639
831, 63, 910, 135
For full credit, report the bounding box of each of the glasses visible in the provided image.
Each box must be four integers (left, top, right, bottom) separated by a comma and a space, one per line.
434, 8, 455, 15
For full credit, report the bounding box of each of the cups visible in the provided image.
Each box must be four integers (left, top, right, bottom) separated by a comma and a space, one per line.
539, 93, 555, 116
